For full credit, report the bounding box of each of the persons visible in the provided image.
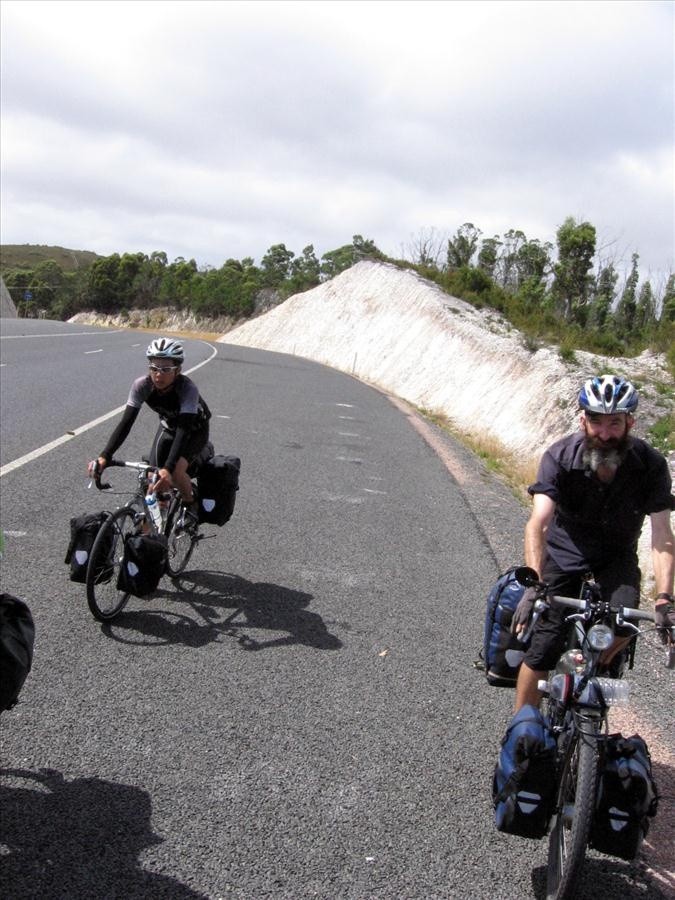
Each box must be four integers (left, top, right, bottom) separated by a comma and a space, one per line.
513, 374, 675, 716
87, 338, 211, 535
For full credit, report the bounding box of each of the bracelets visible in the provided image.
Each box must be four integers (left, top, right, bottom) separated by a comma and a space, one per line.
654, 592, 675, 604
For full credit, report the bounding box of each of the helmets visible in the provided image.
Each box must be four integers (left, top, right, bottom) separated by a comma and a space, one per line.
578, 375, 638, 414
147, 339, 185, 363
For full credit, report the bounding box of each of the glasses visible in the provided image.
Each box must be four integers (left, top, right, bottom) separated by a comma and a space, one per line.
148, 365, 177, 373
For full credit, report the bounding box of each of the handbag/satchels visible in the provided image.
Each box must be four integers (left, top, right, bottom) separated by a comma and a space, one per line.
197, 455, 241, 527
0, 593, 35, 713
116, 533, 170, 600
588, 734, 660, 861
491, 703, 565, 839
65, 514, 115, 584
483, 566, 532, 689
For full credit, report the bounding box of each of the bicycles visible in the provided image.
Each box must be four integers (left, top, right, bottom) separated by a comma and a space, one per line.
86, 459, 200, 623
514, 565, 675, 900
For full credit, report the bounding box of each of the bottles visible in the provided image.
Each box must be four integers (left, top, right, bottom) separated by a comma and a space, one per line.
145, 495, 162, 528
556, 647, 587, 675
538, 673, 630, 705
